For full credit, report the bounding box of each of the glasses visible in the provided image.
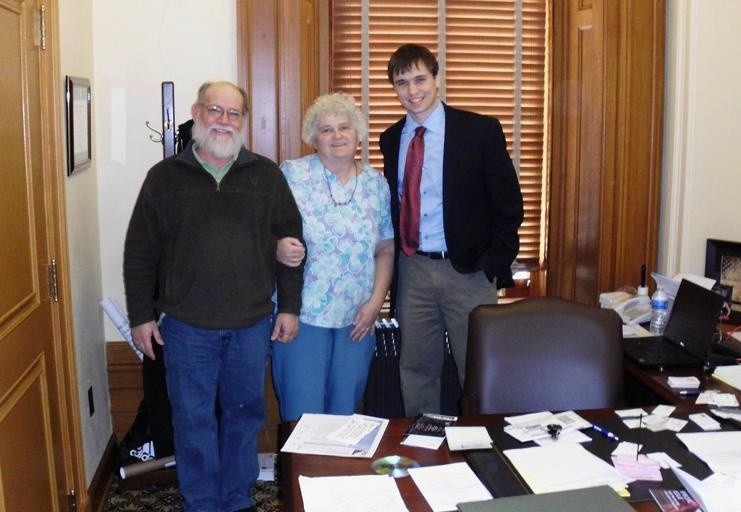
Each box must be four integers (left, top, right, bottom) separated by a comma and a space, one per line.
201, 104, 240, 121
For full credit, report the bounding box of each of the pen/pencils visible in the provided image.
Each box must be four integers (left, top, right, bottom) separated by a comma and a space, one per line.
592, 424, 620, 441
679, 390, 700, 394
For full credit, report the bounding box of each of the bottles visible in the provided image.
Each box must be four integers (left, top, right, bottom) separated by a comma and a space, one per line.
650, 285, 668, 334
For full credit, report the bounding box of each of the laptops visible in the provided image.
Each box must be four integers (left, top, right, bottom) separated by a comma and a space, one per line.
619, 278, 726, 372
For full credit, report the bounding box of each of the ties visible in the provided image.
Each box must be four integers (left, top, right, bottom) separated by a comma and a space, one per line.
398, 126, 426, 257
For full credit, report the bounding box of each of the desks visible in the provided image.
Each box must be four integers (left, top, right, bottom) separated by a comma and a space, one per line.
618, 318, 741, 407
280, 375, 741, 512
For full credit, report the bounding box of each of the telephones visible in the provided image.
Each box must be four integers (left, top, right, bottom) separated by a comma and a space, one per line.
613, 295, 653, 325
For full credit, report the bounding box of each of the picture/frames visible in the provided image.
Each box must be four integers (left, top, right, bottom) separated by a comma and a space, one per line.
66, 73, 93, 178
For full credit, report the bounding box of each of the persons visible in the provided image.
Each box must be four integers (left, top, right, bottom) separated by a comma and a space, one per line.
123, 81, 307, 511
276, 93, 396, 420
378, 43, 525, 415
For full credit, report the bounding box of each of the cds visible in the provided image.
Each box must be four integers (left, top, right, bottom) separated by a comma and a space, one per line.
372, 456, 420, 477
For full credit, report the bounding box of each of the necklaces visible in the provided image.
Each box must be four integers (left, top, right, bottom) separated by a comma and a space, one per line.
325, 161, 358, 206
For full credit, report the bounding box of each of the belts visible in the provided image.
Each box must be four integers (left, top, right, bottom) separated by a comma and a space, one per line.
415, 249, 449, 260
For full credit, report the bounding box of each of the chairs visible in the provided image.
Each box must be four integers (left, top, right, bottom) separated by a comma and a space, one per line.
462, 298, 623, 413
